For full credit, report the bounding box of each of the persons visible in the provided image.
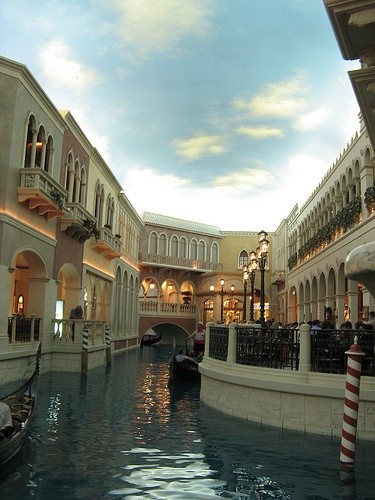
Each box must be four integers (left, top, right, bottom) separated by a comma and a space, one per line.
216, 311, 375, 379
185, 321, 207, 358
0, 401, 14, 438
17, 308, 24, 319
70, 305, 83, 342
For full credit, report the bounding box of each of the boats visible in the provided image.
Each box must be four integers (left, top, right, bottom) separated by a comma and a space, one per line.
168, 354, 201, 391
141, 328, 162, 346
0, 340, 42, 480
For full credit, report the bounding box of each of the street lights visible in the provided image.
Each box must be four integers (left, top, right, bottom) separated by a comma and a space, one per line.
255, 229, 270, 327
242, 263, 249, 324
248, 250, 258, 324
210, 278, 235, 322
